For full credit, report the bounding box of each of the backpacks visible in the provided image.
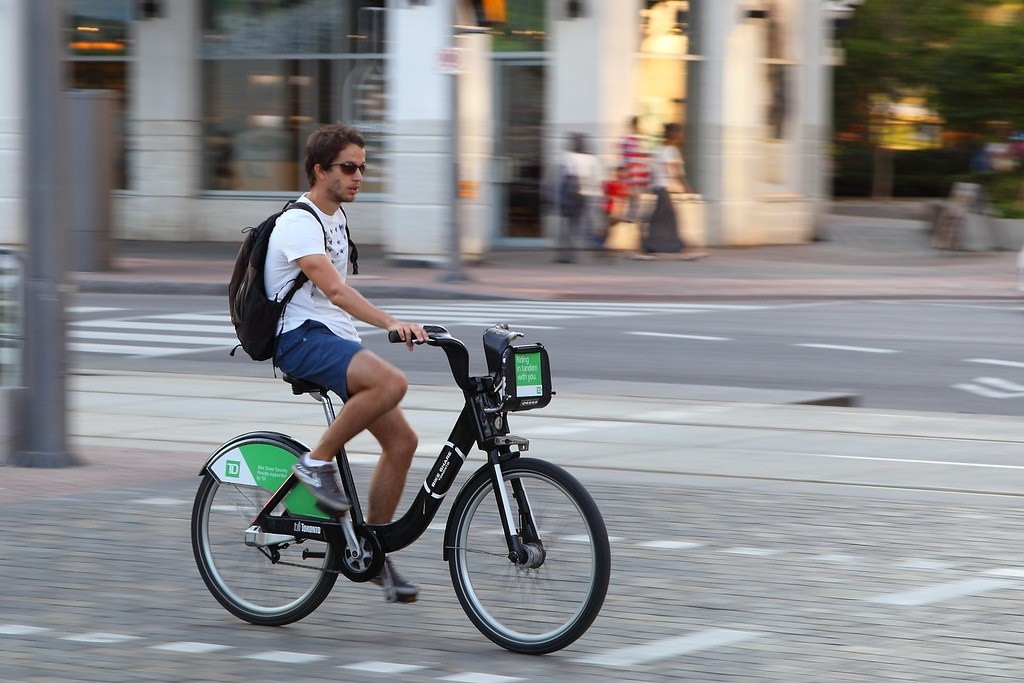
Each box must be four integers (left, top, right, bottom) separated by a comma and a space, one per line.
228, 200, 358, 362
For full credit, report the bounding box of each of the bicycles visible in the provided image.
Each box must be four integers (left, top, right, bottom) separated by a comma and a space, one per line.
190, 325, 612, 658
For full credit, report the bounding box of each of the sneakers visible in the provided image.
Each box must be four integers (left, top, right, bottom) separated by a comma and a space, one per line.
369, 554, 420, 594
290, 452, 351, 511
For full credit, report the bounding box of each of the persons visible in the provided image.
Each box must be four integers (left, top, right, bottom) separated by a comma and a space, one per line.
982, 121, 1024, 171
263, 122, 429, 593
554, 116, 696, 264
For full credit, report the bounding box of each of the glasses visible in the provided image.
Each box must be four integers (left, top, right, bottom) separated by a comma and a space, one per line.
326, 161, 367, 176
